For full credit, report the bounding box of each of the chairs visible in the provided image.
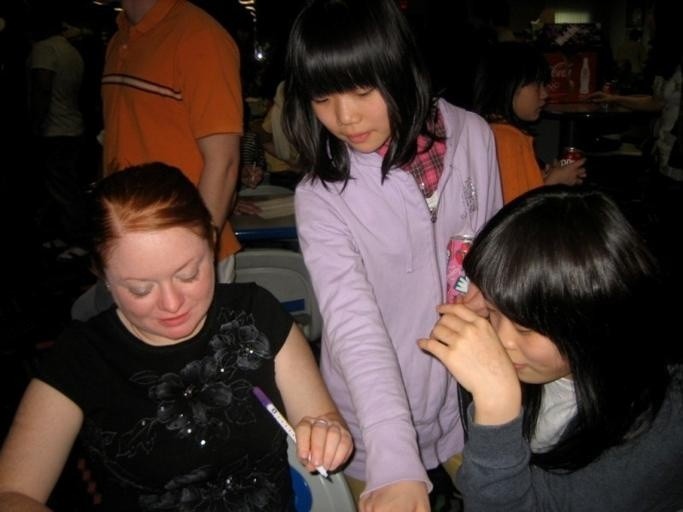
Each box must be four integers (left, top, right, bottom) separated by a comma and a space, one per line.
235, 249, 324, 342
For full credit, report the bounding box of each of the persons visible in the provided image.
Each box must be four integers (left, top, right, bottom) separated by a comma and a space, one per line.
416, 183, 683, 511
1, 161, 354, 512
280, 0, 503, 511
473, 2, 683, 205
25, 2, 305, 284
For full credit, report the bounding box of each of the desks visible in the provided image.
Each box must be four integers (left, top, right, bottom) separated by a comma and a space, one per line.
230, 182, 295, 232
540, 100, 636, 157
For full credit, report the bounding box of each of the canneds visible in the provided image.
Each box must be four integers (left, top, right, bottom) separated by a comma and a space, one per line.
599, 82, 617, 109
447, 235, 477, 304
559, 147, 584, 167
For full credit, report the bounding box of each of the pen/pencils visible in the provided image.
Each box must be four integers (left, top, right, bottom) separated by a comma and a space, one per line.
252, 386, 333, 483
